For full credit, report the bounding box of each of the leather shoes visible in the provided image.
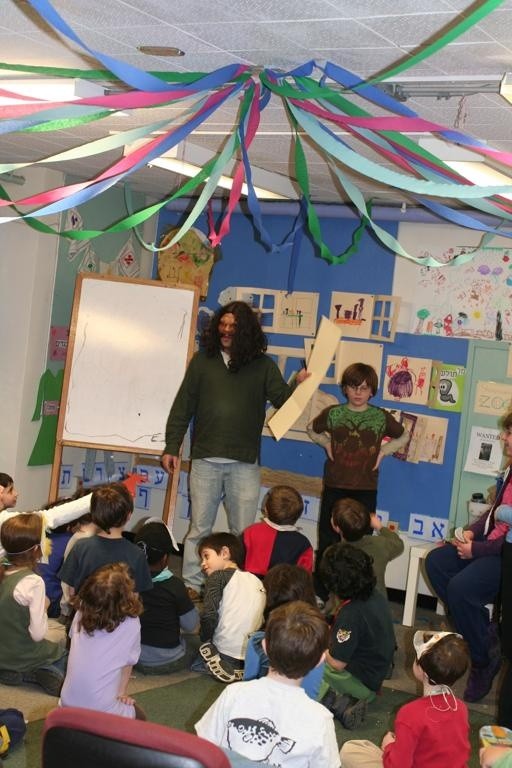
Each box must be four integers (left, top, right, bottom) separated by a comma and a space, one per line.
462, 645, 502, 703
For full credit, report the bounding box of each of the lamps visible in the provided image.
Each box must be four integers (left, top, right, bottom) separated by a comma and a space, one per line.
2, 78, 107, 101
418, 139, 511, 202
123, 138, 308, 200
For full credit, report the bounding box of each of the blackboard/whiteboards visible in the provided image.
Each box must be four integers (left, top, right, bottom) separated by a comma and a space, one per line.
56, 273, 200, 459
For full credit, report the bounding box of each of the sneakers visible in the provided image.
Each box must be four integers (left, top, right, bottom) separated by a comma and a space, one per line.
32, 668, 63, 690
342, 698, 367, 730
0, 670, 23, 686
331, 694, 352, 718
186, 587, 204, 602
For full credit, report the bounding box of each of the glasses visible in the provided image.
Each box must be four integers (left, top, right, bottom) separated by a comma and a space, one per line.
345, 384, 370, 392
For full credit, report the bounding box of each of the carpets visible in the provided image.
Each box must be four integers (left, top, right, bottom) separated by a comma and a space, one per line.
1, 674, 511, 768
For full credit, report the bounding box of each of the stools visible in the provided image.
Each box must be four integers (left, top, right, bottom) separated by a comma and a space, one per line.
401, 540, 494, 628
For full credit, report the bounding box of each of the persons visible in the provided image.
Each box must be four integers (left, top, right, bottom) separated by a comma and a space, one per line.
341, 631, 470, 767
307, 362, 410, 605
263, 565, 379, 716
0, 473, 90, 533
65, 484, 152, 596
0, 513, 66, 673
163, 302, 312, 603
280, 701, 310, 702
194, 603, 340, 766
194, 532, 266, 682
235, 486, 315, 581
60, 564, 146, 723
320, 497, 404, 604
424, 413, 510, 702
130, 518, 200, 674
321, 543, 398, 694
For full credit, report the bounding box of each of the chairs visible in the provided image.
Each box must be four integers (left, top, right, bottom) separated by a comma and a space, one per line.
39, 706, 232, 768
